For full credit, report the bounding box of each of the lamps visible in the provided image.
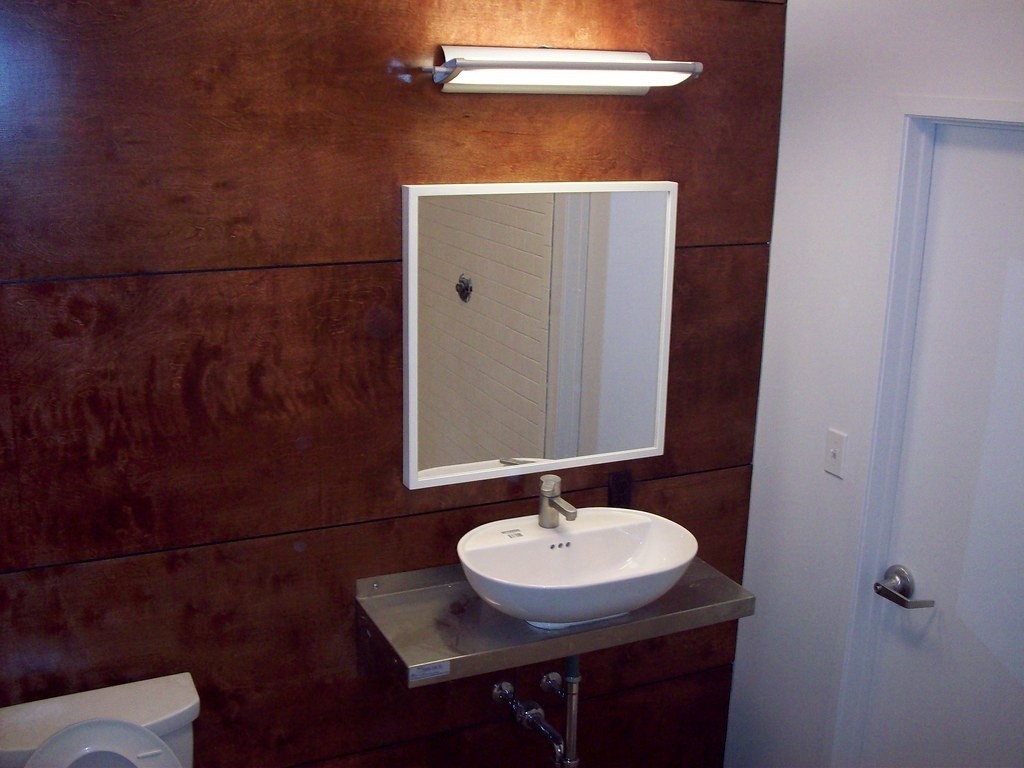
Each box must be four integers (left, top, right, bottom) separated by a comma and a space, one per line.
413, 45, 703, 96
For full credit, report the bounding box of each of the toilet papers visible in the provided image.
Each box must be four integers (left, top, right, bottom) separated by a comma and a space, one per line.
431, 41, 698, 102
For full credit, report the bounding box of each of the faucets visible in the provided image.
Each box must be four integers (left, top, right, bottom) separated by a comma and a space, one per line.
499, 456, 542, 466
536, 472, 579, 530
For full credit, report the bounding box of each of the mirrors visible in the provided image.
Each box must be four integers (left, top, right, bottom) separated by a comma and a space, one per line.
403, 180, 678, 491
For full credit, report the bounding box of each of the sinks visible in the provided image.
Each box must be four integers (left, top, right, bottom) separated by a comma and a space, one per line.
418, 457, 555, 479
456, 506, 699, 630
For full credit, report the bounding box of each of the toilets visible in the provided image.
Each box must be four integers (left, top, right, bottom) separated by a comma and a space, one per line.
0, 671, 201, 768
